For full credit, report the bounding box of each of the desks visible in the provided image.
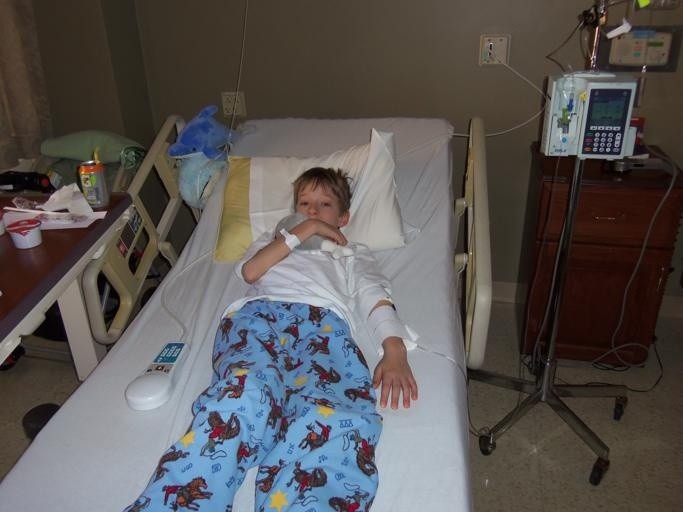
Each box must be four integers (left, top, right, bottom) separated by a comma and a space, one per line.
0, 192, 133, 382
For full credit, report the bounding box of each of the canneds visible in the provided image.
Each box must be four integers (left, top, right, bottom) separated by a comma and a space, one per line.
78, 160, 110, 208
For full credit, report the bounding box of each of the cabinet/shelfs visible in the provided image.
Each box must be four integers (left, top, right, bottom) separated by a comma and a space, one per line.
514, 143, 681, 365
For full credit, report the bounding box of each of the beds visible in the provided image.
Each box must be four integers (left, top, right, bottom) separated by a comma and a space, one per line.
0, 117, 491, 511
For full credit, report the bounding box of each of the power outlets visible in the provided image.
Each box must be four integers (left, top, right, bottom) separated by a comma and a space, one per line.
219, 91, 247, 119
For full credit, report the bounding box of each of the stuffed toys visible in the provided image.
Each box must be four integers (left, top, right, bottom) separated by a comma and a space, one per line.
273, 213, 354, 260
167, 105, 235, 210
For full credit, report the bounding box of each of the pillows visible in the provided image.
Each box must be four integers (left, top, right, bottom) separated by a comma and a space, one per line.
213, 127, 410, 262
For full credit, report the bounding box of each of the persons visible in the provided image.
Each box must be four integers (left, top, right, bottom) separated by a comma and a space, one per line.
124, 166, 418, 512
